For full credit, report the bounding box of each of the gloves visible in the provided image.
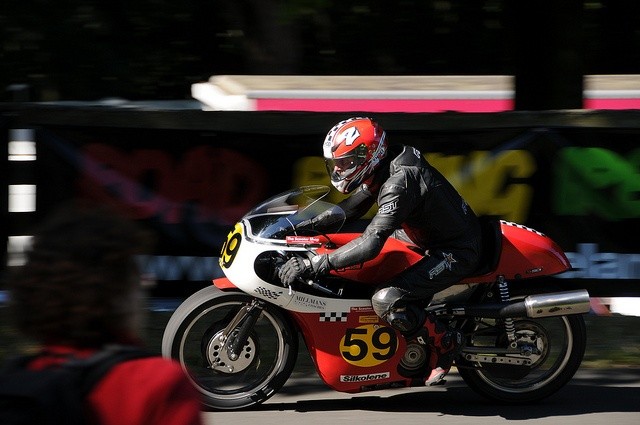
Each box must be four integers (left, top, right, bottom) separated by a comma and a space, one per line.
278, 255, 314, 288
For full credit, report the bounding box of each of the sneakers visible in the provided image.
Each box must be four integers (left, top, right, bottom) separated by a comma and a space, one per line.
423, 313, 463, 387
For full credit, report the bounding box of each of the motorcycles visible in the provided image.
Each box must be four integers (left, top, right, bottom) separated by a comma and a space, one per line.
162, 194, 595, 413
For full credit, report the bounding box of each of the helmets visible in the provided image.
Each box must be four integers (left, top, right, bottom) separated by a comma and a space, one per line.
323, 117, 389, 195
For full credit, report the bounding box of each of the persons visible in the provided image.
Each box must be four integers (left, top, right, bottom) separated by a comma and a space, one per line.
0, 207, 203, 424
278, 116, 483, 387
